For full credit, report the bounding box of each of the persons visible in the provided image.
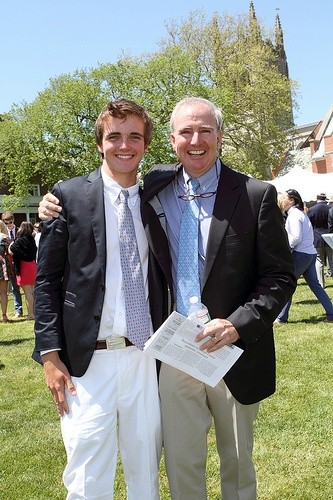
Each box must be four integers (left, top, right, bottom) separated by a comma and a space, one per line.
9, 222, 36, 320
37, 98, 296, 499
31, 99, 165, 500
2, 212, 23, 317
305, 192, 333, 288
272, 190, 333, 323
0, 220, 12, 321
34, 223, 41, 264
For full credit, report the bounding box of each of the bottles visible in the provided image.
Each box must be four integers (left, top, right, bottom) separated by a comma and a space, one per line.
188, 297, 211, 325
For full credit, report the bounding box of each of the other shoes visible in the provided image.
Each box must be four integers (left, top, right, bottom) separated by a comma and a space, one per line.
14, 311, 23, 317
2, 316, 9, 322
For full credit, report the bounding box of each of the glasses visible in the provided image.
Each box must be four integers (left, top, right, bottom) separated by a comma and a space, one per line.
175, 163, 218, 201
4, 221, 13, 224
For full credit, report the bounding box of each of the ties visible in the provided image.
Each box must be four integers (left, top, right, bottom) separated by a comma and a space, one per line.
9, 231, 14, 240
116, 190, 150, 353
177, 179, 201, 317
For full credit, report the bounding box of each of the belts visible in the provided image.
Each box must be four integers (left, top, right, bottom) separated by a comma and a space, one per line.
94, 337, 150, 351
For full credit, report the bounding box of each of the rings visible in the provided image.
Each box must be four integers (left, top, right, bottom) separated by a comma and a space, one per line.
214, 337, 218, 341
